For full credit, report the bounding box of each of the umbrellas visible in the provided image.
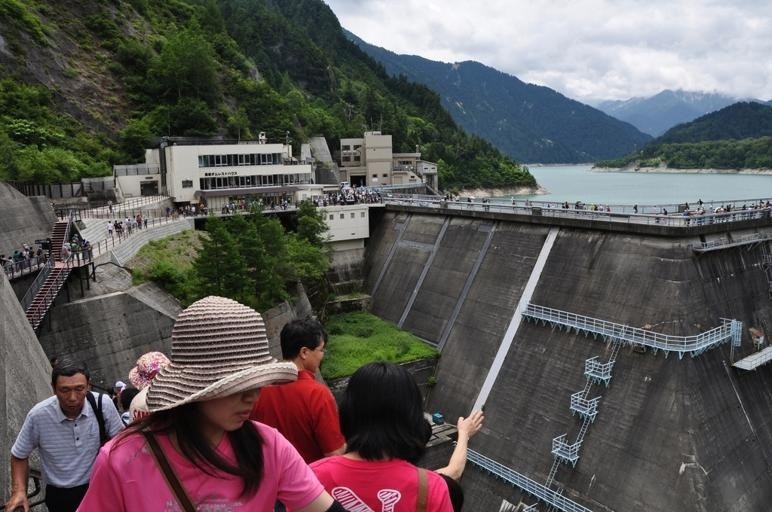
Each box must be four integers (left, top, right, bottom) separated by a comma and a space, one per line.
576, 201, 580, 204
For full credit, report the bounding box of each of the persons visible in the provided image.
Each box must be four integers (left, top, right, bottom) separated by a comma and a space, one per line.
107, 214, 147, 237
684, 197, 771, 226
0, 233, 91, 274
525, 199, 528, 205
466, 198, 471, 208
306, 360, 457, 512
582, 203, 586, 214
78, 295, 350, 512
662, 208, 667, 225
594, 205, 597, 211
561, 203, 565, 212
606, 205, 610, 211
529, 201, 532, 206
450, 192, 452, 200
446, 193, 449, 199
430, 405, 485, 481
469, 199, 473, 207
3, 349, 171, 512
435, 472, 465, 512
565, 201, 568, 213
456, 195, 459, 200
107, 199, 113, 213
590, 205, 592, 211
575, 203, 579, 214
598, 204, 603, 211
633, 205, 638, 213
487, 198, 490, 209
253, 318, 348, 512
164, 185, 384, 220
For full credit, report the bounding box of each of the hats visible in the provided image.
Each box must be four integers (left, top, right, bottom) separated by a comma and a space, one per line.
128, 351, 171, 392
146, 294, 298, 414
